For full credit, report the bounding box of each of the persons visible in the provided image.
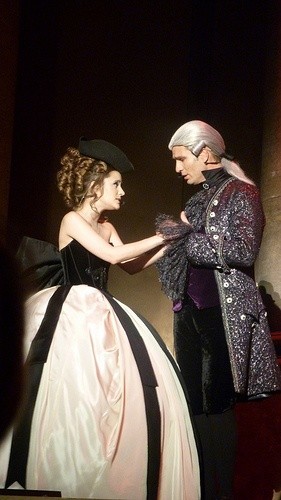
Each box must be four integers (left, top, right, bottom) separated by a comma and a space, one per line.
0, 146, 201, 500
156, 119, 281, 500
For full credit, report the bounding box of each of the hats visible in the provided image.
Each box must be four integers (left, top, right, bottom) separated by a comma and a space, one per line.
79, 135, 135, 173
